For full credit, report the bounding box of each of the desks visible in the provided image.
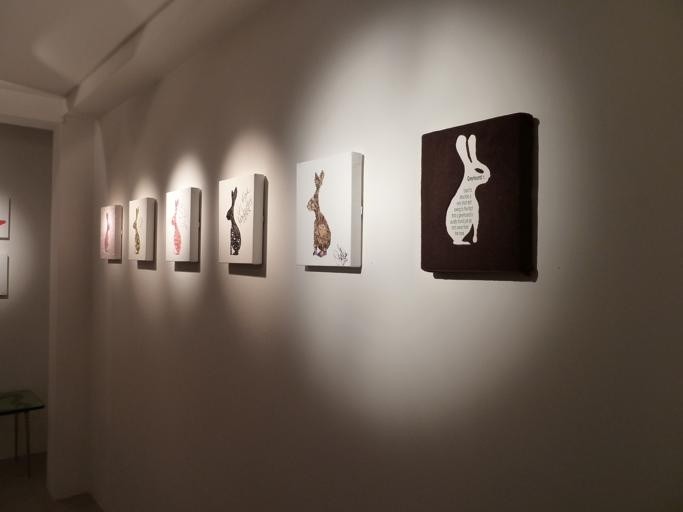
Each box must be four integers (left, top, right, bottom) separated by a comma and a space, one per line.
0, 388, 45, 481
